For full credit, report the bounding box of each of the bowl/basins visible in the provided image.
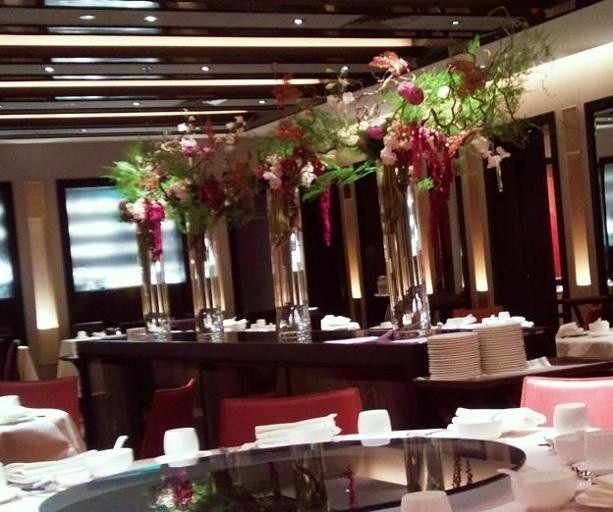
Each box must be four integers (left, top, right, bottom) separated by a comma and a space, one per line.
505, 471, 575, 511
455, 420, 502, 440
588, 322, 609, 335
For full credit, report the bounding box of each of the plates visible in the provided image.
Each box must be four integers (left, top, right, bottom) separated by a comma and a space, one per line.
574, 491, 613, 509
426, 320, 525, 381
578, 462, 612, 473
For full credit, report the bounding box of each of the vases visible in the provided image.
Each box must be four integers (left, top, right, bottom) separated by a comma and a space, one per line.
136, 222, 171, 332
377, 160, 431, 339
183, 215, 223, 332
267, 188, 310, 338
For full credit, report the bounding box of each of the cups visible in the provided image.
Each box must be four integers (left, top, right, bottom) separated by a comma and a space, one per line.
400, 489, 452, 512
88, 447, 133, 477
356, 409, 392, 448
584, 431, 613, 465
0, 394, 20, 419
553, 433, 585, 463
163, 427, 199, 468
553, 403, 587, 435
